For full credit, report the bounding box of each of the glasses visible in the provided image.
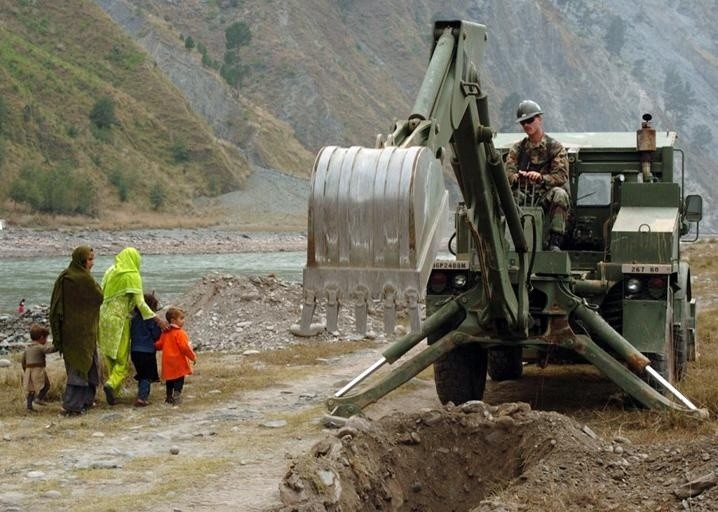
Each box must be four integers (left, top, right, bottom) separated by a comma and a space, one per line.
520, 117, 535, 124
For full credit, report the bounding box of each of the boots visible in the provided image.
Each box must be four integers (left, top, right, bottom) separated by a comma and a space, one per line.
547, 229, 564, 250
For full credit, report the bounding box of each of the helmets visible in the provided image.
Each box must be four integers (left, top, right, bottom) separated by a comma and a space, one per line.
513, 100, 545, 124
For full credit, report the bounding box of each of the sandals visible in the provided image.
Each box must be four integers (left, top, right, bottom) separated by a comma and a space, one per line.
135, 399, 151, 406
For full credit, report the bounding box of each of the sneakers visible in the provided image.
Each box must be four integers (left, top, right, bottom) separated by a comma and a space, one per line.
103, 383, 115, 406
164, 395, 183, 404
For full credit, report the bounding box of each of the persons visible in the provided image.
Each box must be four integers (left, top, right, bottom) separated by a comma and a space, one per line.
20, 325, 60, 413
502, 98, 573, 253
153, 308, 196, 404
97, 246, 171, 405
129, 294, 162, 407
15, 298, 26, 314
48, 244, 104, 416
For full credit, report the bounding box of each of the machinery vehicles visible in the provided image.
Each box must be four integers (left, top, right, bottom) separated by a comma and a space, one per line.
300, 20, 710, 423
